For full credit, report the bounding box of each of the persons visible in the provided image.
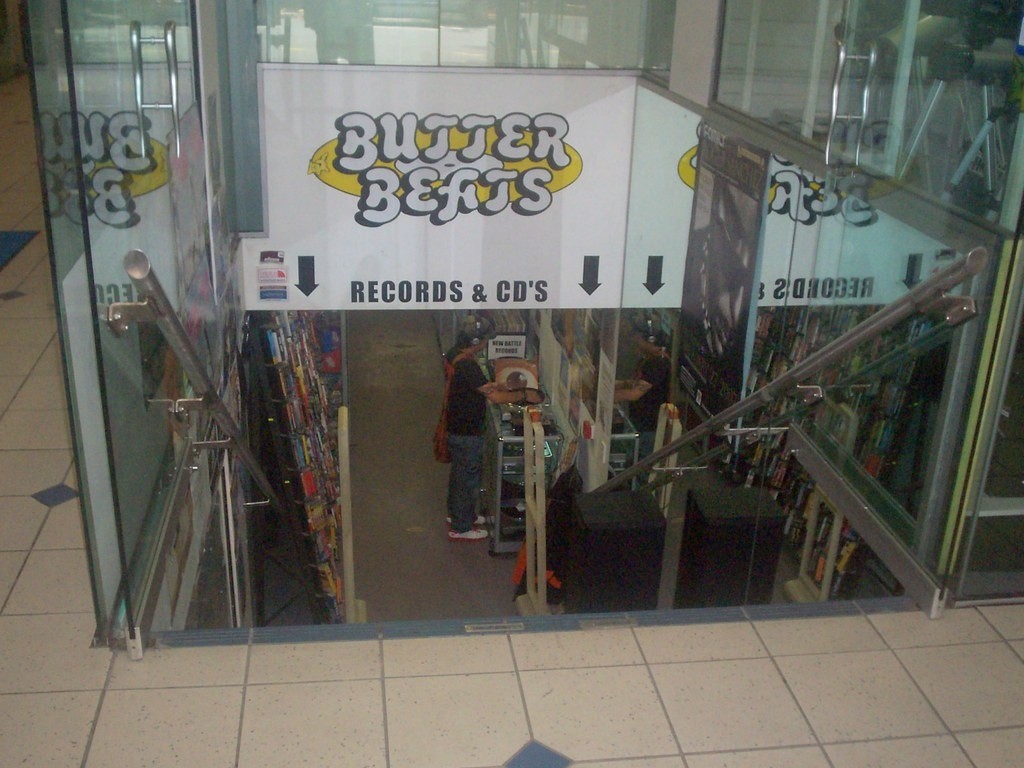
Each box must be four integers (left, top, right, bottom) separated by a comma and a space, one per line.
615, 329, 672, 484
443, 314, 543, 539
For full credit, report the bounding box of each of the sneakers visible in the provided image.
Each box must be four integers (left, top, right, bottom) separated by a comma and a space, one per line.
446, 514, 486, 527
447, 527, 488, 542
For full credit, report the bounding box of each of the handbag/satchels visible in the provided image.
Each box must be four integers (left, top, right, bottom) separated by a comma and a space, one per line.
433, 413, 448, 463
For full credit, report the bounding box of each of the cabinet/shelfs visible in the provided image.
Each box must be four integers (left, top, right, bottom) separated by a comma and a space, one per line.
481, 386, 563, 553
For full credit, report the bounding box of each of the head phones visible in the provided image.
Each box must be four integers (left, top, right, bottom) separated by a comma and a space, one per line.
647, 320, 656, 342
471, 317, 482, 346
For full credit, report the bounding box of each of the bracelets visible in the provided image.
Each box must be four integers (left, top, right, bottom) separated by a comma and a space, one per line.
522, 389, 527, 401
